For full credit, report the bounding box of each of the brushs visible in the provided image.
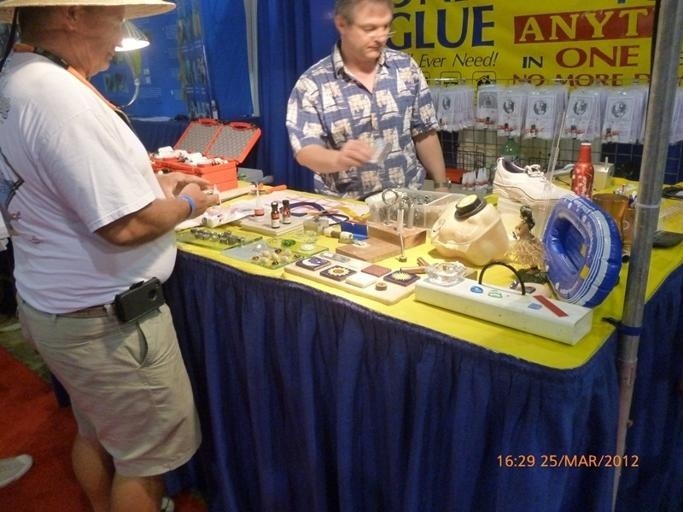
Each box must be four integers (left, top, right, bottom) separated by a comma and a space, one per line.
369, 139, 393, 164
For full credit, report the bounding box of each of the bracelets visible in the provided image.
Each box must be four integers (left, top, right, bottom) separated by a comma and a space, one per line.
177, 192, 196, 221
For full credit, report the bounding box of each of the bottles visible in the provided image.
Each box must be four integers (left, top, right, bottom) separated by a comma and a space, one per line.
571, 142, 594, 202
281, 199, 290, 223
270, 201, 280, 228
503, 135, 520, 168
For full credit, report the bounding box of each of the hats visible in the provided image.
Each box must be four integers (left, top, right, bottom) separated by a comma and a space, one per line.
0, 0, 179, 24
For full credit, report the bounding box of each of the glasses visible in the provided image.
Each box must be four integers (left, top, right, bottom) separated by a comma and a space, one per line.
345, 19, 398, 41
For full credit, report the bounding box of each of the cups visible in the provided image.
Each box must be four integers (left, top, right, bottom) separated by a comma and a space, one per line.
591, 193, 628, 241
302, 221, 319, 243
622, 207, 634, 251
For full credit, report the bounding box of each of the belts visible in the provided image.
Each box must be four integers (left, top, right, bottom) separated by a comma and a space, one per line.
55, 305, 109, 319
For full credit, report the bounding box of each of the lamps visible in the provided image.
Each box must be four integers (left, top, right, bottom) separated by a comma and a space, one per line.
111, 17, 151, 54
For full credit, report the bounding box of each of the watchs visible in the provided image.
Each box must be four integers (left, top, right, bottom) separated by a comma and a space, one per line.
434, 177, 453, 189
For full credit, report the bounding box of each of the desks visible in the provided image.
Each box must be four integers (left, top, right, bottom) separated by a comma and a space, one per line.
137, 163, 682, 511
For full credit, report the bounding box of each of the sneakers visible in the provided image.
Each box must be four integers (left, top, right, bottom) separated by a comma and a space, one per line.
491, 153, 578, 209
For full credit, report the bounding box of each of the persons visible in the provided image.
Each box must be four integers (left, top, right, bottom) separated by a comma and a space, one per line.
0, 452, 34, 488
0, 0, 221, 512
285, 0, 454, 199
